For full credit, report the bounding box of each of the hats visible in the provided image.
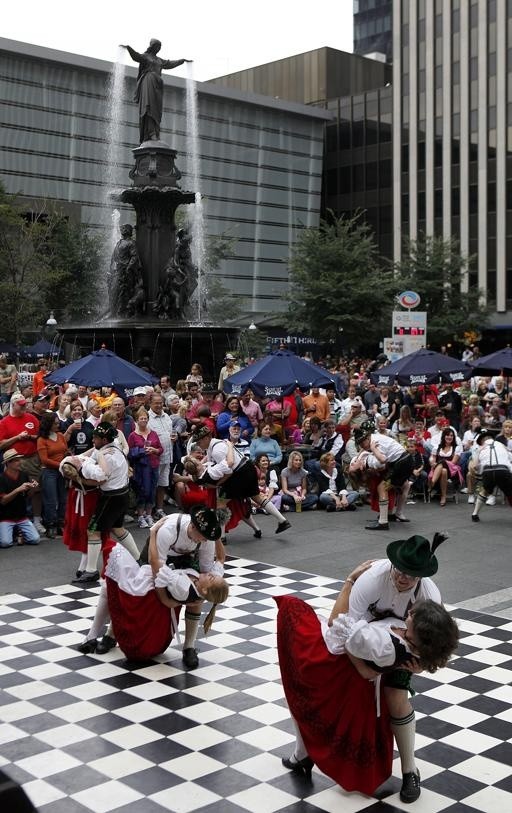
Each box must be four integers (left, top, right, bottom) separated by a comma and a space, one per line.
1, 359, 59, 463
355, 428, 370, 445
189, 504, 221, 541
476, 428, 501, 445
387, 535, 438, 577
200, 384, 219, 396
192, 424, 211, 443
133, 386, 147, 396
90, 420, 118, 442
224, 354, 236, 362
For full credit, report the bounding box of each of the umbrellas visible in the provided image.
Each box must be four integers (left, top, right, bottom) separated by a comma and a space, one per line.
26, 338, 64, 359
0, 343, 21, 363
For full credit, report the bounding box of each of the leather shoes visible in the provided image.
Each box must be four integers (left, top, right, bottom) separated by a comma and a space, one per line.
98, 635, 116, 654
71, 568, 100, 582
401, 768, 421, 803
365, 512, 410, 529
254, 530, 263, 539
274, 520, 291, 534
183, 648, 200, 668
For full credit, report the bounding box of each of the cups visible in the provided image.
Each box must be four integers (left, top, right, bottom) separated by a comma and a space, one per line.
143, 440, 152, 448
73, 418, 81, 429
171, 428, 177, 440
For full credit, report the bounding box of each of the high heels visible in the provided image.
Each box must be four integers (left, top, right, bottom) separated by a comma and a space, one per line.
77, 638, 98, 653
283, 755, 314, 774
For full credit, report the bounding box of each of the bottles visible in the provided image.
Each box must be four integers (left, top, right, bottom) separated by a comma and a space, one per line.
296, 501, 301, 512
17, 528, 24, 545
313, 399, 317, 412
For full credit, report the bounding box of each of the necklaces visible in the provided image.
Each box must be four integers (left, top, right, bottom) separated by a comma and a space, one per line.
401, 627, 417, 648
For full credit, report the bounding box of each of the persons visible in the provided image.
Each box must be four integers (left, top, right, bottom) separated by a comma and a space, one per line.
158, 228, 198, 317
264, 595, 459, 779
1, 358, 66, 415
348, 531, 451, 801
80, 540, 228, 658
1, 383, 512, 534
2, 447, 40, 546
302, 352, 388, 397
160, 353, 256, 416
61, 424, 143, 582
97, 507, 223, 669
184, 426, 292, 543
461, 349, 512, 419
106, 222, 144, 321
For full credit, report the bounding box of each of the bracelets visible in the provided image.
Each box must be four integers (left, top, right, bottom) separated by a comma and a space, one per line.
347, 577, 355, 584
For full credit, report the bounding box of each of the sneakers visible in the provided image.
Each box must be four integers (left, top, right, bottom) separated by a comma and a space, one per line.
252, 496, 370, 514
125, 495, 178, 527
440, 488, 497, 522
